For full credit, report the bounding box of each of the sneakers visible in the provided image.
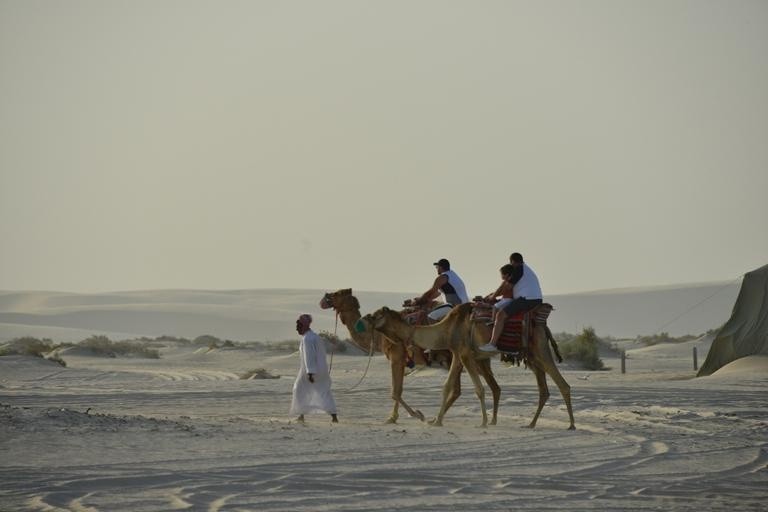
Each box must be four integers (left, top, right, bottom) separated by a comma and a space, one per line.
478, 344, 498, 352
486, 320, 495, 326
424, 349, 432, 353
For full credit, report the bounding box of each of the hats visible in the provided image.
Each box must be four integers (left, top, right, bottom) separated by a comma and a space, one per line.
299, 315, 312, 334
433, 258, 450, 266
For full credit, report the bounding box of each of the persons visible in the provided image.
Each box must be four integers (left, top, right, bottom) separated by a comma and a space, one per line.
409, 259, 469, 354
486, 264, 516, 326
289, 313, 339, 426
478, 253, 544, 351
